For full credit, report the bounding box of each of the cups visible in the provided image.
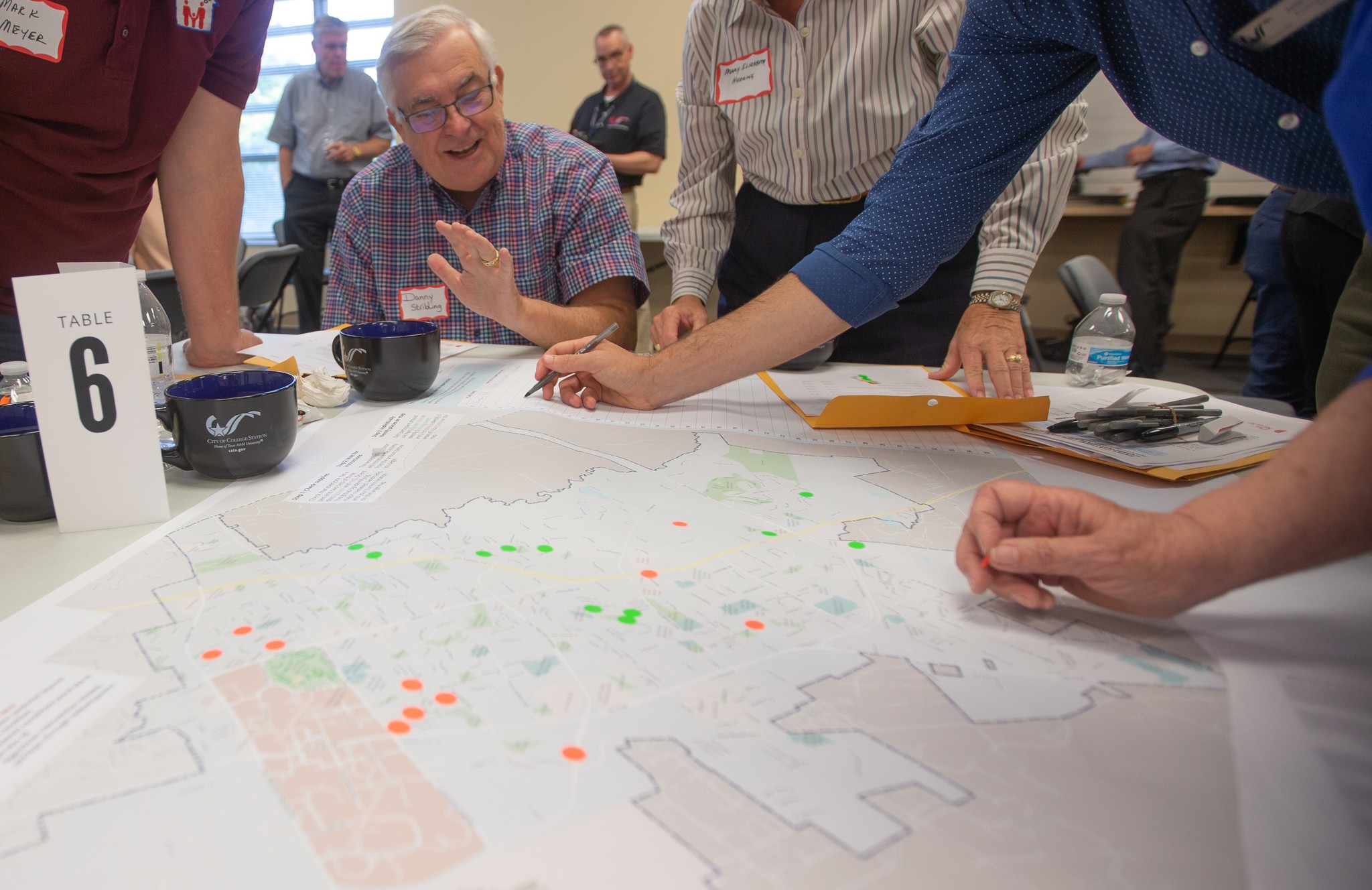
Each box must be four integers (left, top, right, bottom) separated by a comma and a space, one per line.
320, 134, 336, 154
332, 320, 441, 401
0, 401, 57, 522
155, 370, 298, 479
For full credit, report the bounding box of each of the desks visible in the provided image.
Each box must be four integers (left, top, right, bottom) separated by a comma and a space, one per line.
1063, 200, 1261, 369
0, 333, 1372, 890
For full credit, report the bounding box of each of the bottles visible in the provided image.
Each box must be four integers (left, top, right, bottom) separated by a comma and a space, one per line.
135, 269, 175, 471
1065, 293, 1136, 387
0, 361, 34, 405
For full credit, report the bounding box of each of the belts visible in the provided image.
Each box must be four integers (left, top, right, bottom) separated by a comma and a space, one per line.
293, 172, 352, 189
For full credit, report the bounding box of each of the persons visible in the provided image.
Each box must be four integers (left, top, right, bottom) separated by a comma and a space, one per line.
650, 2, 1087, 402
1074, 126, 1216, 381
570, 26, 666, 232
279, 17, 393, 336
0, 0, 274, 371
317, 3, 642, 362
534, 2, 1371, 623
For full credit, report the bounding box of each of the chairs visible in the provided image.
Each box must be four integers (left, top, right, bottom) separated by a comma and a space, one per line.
145, 220, 332, 345
1061, 256, 1134, 323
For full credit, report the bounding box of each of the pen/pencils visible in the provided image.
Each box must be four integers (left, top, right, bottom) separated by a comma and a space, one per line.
523, 321, 620, 400
1048, 394, 1222, 441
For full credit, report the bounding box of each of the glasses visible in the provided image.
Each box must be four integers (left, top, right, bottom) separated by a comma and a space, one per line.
594, 49, 622, 66
396, 69, 495, 134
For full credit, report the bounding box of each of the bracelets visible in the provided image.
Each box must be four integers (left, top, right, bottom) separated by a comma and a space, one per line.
353, 143, 363, 157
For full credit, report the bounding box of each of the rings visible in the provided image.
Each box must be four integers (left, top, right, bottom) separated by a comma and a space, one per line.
1005, 354, 1023, 363
480, 249, 500, 267
653, 343, 661, 353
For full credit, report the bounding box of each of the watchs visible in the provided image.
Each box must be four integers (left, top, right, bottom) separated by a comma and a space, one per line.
969, 290, 1022, 313
